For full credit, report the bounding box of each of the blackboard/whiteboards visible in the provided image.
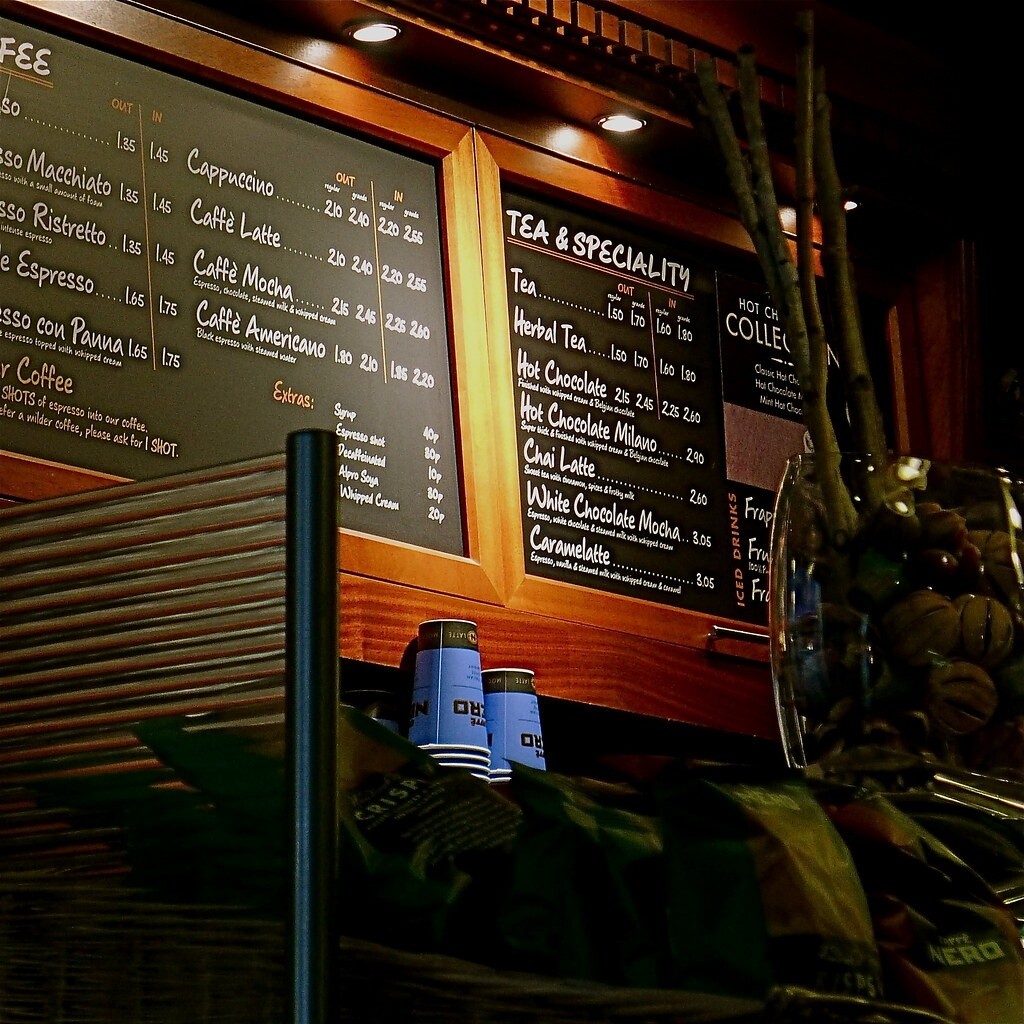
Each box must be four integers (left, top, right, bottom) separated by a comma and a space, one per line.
1, 0, 894, 736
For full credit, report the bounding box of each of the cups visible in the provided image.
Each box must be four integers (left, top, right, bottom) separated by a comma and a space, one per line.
342, 690, 401, 736
408, 614, 547, 785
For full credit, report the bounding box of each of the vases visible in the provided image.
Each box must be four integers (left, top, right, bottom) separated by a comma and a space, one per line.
763, 454, 1024, 810
